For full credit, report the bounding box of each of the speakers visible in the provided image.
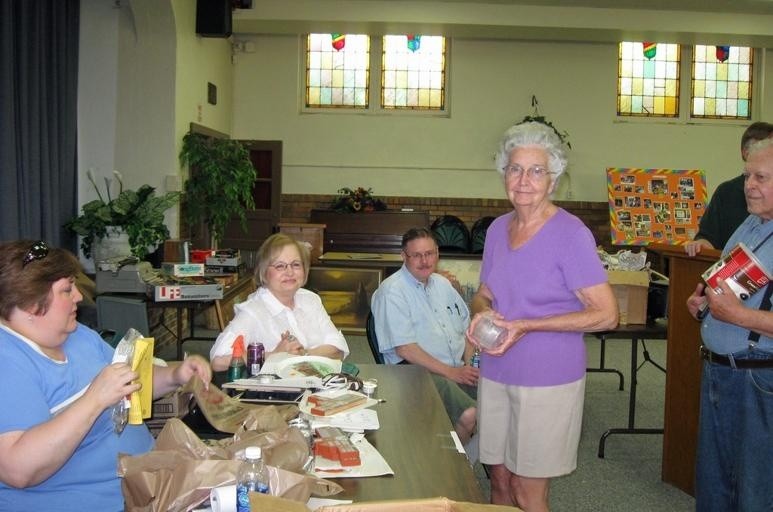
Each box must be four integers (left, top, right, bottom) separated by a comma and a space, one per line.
196, 0, 232, 37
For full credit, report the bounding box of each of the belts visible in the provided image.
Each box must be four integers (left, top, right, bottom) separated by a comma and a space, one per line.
703, 349, 772, 369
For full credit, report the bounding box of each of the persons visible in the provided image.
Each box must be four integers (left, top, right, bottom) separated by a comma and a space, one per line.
209, 233, 350, 372
684, 121, 773, 257
463, 121, 620, 512
687, 138, 773, 512
0, 240, 211, 512
371, 228, 480, 446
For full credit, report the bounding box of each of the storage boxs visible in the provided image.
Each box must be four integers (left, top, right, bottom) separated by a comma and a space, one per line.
606, 271, 650, 326
145, 391, 189, 438
277, 222, 328, 266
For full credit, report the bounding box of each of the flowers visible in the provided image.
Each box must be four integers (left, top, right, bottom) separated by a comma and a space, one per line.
328, 188, 386, 214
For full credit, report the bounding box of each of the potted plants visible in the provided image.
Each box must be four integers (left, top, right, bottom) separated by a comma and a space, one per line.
72, 165, 185, 272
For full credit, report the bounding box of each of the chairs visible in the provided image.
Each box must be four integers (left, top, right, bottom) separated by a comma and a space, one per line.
469, 216, 499, 255
429, 213, 470, 255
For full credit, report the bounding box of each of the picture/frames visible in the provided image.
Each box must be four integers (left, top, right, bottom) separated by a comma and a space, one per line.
302, 265, 385, 336
213, 275, 256, 331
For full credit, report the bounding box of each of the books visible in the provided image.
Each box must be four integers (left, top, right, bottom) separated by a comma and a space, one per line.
221, 352, 395, 480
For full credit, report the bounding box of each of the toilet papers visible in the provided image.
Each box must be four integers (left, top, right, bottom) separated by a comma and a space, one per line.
210, 485, 237, 512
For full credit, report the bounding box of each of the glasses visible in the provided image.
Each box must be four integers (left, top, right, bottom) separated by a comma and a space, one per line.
507, 166, 553, 178
402, 249, 437, 259
268, 261, 303, 271
23, 240, 49, 268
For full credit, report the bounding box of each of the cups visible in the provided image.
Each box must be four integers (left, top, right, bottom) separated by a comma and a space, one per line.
470, 316, 508, 351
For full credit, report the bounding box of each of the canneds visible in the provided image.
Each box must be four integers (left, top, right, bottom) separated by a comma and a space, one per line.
246, 341, 265, 377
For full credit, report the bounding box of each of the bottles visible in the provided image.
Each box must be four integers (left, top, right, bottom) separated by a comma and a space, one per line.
235, 446, 269, 512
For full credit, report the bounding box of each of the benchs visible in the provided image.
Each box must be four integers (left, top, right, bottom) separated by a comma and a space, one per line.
366, 311, 410, 364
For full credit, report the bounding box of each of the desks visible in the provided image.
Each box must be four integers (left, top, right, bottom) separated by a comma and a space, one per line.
148, 268, 256, 360
647, 241, 723, 497
584, 321, 667, 458
433, 252, 483, 318
212, 362, 489, 512
